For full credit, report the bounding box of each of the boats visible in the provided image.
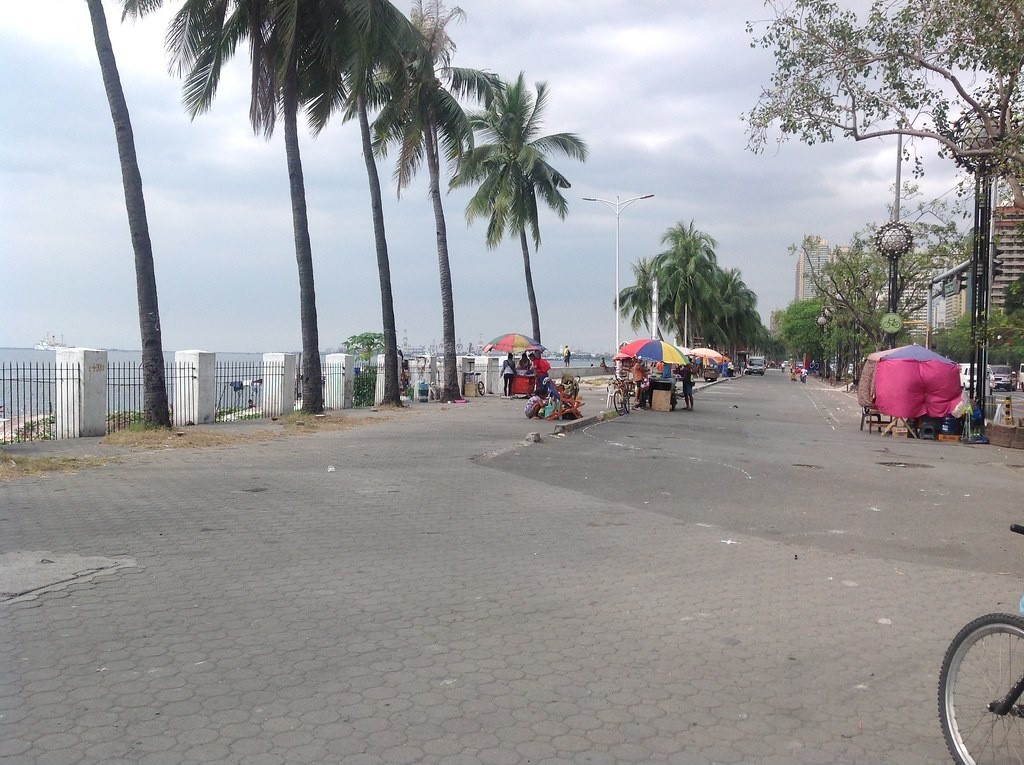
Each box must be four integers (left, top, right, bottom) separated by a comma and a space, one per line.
35, 333, 75, 351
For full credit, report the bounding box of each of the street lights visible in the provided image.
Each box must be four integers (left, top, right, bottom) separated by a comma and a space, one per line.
874, 223, 913, 349
582, 194, 656, 377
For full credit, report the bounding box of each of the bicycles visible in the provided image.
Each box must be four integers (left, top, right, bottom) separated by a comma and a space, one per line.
610, 374, 632, 416
463, 371, 485, 396
937, 524, 1024, 765
606, 374, 618, 393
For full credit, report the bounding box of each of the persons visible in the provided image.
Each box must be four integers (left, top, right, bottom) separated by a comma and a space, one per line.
591, 364, 593, 366
781, 361, 808, 381
499, 353, 516, 396
663, 363, 671, 378
398, 349, 407, 389
524, 388, 548, 419
722, 360, 745, 377
655, 360, 664, 374
515, 351, 532, 369
600, 357, 609, 372
681, 363, 700, 411
633, 356, 650, 403
528, 354, 549, 397
564, 345, 570, 367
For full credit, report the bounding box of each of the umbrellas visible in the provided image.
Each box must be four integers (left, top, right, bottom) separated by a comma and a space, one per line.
691, 357, 719, 366
482, 333, 548, 364
675, 346, 697, 356
689, 348, 722, 364
723, 356, 731, 362
621, 339, 691, 367
612, 354, 633, 360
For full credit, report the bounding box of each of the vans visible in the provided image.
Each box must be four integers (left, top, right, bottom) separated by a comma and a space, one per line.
1019, 364, 1024, 392
958, 363, 996, 395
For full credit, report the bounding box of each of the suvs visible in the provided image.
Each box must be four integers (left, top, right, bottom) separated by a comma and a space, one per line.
990, 365, 1018, 392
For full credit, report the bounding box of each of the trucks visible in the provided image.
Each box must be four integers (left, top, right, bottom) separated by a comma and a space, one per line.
746, 355, 765, 376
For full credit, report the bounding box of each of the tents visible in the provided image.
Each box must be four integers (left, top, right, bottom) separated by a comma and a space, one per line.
857, 343, 962, 418
